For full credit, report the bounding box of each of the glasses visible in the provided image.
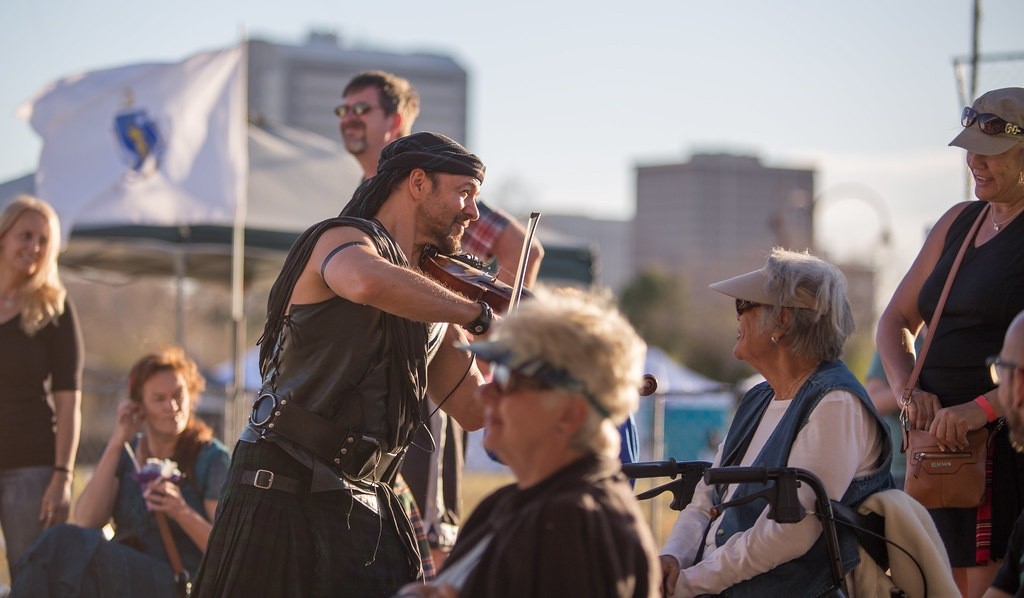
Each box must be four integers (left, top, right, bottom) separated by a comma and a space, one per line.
960, 107, 1024, 136
986, 357, 1024, 384
489, 359, 520, 394
334, 102, 372, 117
735, 298, 764, 315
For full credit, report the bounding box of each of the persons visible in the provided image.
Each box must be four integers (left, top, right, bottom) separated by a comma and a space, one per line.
188, 132, 503, 598
6, 347, 233, 598
0, 197, 85, 582
399, 286, 663, 598
658, 248, 895, 598
866, 87, 1024, 598
337, 71, 547, 555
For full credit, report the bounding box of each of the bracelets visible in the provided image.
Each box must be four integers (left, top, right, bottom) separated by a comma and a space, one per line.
974, 394, 997, 423
463, 301, 493, 336
54, 465, 73, 474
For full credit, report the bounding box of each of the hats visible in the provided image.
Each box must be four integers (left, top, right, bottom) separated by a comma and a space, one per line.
454, 336, 611, 420
709, 264, 832, 315
948, 87, 1024, 156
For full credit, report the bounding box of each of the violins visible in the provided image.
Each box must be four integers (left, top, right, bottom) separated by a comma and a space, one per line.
420, 241, 657, 395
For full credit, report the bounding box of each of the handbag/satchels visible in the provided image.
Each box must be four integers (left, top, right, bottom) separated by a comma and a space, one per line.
899, 416, 1006, 508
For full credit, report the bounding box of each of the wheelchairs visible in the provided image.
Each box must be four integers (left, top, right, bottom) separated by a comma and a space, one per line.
620, 457, 929, 598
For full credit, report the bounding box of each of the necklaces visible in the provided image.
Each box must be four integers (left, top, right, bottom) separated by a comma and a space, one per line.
989, 201, 1024, 231
778, 367, 816, 400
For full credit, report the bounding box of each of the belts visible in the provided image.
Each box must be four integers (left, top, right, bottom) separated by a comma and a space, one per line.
247, 387, 408, 485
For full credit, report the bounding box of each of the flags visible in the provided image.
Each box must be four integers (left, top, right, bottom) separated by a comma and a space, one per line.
28, 45, 241, 231
